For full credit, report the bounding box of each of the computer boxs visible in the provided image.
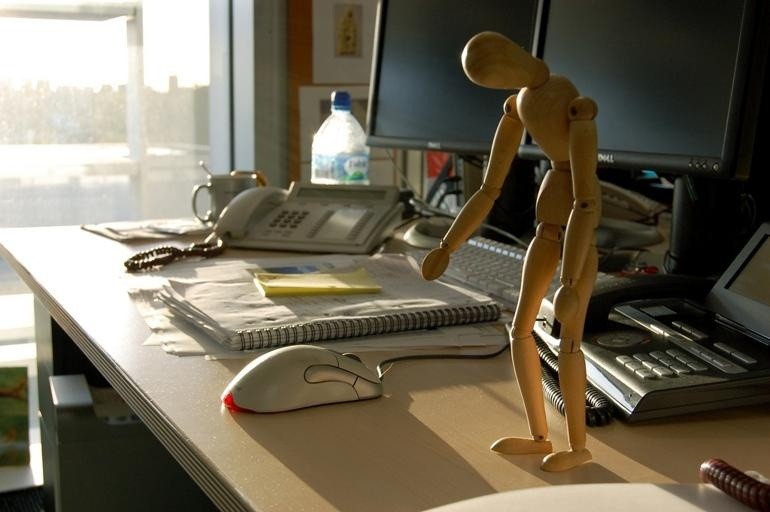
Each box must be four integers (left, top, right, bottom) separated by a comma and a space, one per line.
47, 373, 219, 512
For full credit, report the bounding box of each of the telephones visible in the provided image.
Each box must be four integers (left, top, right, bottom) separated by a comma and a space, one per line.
533, 220, 770, 424
212, 181, 409, 254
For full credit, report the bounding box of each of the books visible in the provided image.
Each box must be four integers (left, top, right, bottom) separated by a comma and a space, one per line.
157, 253, 501, 352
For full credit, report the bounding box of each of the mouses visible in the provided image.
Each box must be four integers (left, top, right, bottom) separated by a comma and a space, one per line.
221, 344, 383, 416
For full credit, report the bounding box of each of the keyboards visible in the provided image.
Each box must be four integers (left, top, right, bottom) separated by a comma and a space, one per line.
407, 233, 615, 316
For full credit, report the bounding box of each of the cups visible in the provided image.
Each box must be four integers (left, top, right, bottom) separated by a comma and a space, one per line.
190, 172, 257, 227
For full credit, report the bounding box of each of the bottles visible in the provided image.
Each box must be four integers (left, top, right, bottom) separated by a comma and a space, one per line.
309, 90, 371, 186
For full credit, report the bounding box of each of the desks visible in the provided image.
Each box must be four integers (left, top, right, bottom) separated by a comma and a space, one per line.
0, 223, 769, 512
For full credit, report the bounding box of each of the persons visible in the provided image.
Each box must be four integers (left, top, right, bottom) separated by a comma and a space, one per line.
422, 32, 604, 476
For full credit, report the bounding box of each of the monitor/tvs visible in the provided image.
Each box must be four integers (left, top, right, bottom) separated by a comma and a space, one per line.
518, 0, 770, 180
364, 0, 538, 155
